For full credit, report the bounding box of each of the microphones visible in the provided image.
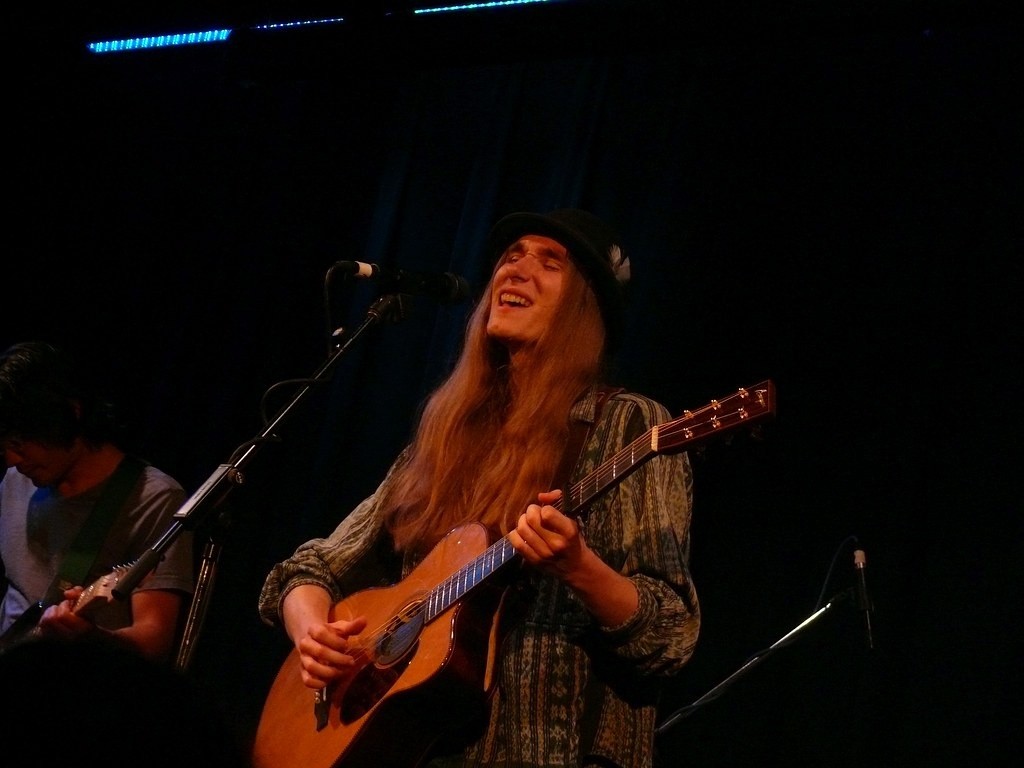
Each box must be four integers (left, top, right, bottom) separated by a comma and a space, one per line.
852, 545, 885, 661
334, 260, 472, 304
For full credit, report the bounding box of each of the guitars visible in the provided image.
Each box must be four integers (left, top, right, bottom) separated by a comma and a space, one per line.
0, 555, 154, 657
249, 379, 772, 768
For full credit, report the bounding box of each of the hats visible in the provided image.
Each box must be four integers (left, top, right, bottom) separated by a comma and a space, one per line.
489, 206, 635, 341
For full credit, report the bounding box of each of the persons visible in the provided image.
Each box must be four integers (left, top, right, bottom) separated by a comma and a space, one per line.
1, 362, 197, 666
255, 203, 702, 768
